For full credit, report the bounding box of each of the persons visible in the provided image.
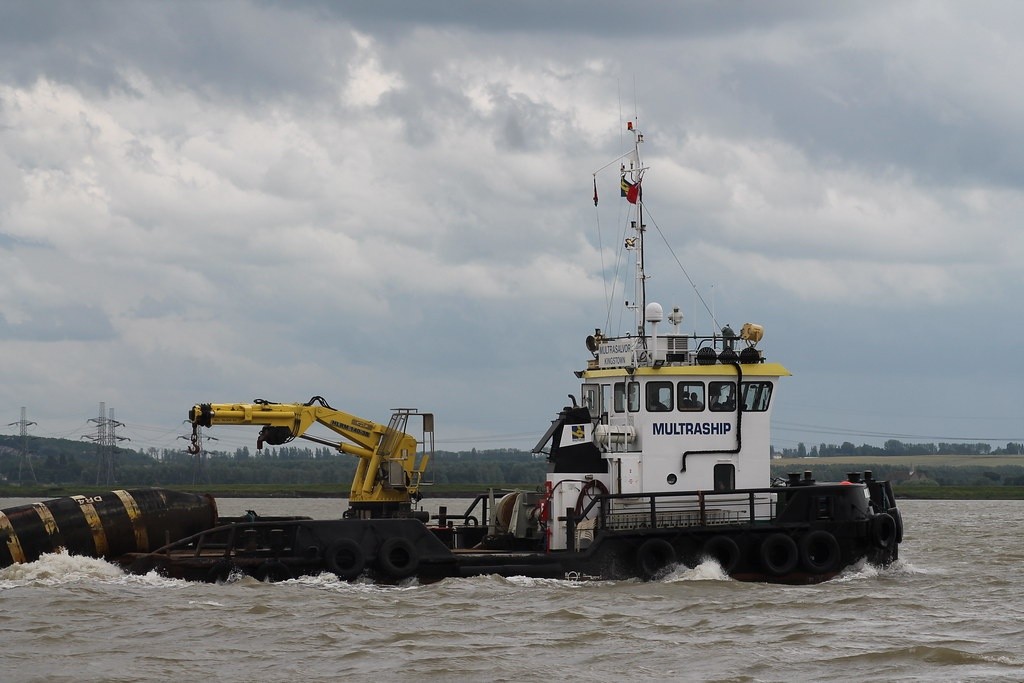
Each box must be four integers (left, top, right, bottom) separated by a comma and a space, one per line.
691, 392, 702, 407
649, 392, 667, 410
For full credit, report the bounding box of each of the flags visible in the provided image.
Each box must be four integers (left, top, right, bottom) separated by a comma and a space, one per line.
621, 179, 632, 197
627, 183, 639, 205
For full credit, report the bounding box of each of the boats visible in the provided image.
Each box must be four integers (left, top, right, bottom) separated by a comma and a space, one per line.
0, 120, 905, 587
